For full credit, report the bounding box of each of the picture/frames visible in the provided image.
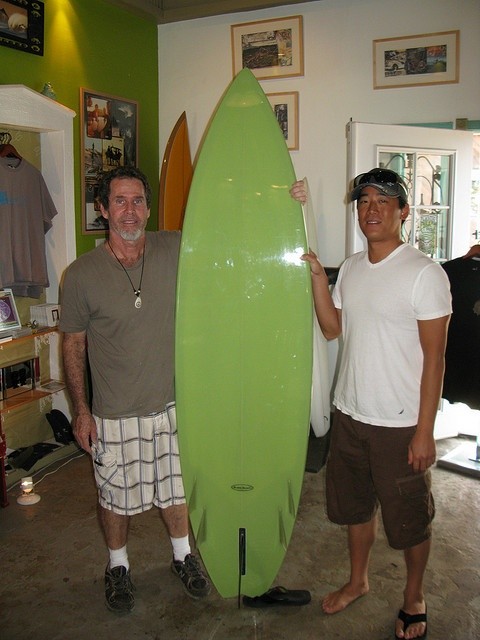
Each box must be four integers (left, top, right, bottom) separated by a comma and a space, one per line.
0, 0, 45, 57
0, 288, 23, 332
79, 86, 140, 236
230, 14, 305, 81
264, 89, 299, 151
372, 29, 460, 89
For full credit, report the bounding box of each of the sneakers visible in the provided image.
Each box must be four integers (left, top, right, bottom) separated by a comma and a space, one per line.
171, 554, 212, 600
104, 560, 136, 614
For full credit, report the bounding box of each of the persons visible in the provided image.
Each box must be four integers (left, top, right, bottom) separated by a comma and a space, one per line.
60, 167, 308, 614
91, 104, 100, 122
302, 166, 453, 637
101, 106, 110, 128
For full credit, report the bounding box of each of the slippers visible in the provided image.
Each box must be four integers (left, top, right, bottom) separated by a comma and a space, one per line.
396, 604, 427, 640
242, 586, 311, 608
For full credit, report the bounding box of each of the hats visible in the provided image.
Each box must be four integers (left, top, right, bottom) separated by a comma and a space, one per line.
350, 168, 408, 204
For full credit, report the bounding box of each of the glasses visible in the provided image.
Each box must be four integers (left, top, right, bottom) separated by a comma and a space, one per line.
353, 171, 397, 187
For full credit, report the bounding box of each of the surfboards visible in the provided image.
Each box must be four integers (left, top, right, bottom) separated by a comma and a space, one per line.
174, 67, 313, 596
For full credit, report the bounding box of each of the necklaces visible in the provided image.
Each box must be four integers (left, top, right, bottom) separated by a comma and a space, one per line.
101, 237, 147, 308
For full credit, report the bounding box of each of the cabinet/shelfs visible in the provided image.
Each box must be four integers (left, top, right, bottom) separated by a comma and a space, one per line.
0, 323, 68, 415
